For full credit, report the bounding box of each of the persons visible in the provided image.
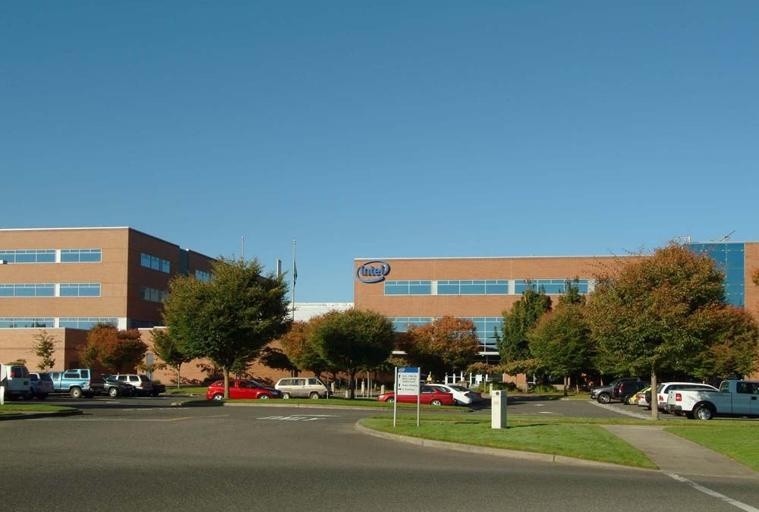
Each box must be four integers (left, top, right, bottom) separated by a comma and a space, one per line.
588, 379, 595, 388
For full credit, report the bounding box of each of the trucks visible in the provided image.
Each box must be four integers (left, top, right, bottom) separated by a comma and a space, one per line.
48, 369, 92, 399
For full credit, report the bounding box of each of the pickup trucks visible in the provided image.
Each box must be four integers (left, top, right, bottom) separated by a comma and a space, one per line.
667, 378, 759, 422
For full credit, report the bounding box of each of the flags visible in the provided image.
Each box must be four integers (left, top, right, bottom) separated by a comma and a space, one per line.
294, 261, 298, 285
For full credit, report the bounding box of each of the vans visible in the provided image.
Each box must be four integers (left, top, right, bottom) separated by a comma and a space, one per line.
104, 374, 154, 398
6, 365, 33, 401
274, 376, 333, 400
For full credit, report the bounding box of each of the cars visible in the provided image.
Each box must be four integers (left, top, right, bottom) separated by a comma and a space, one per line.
31, 372, 57, 401
655, 382, 719, 414
425, 383, 481, 406
90, 374, 137, 399
377, 383, 455, 407
207, 379, 282, 401
591, 377, 660, 410
